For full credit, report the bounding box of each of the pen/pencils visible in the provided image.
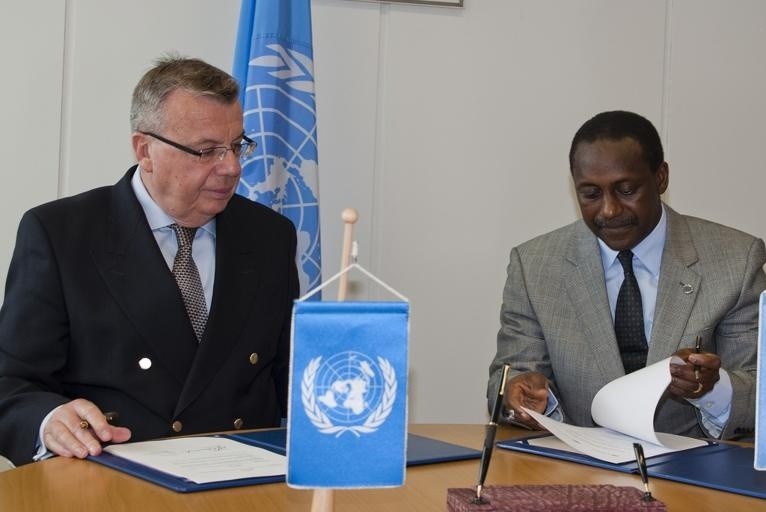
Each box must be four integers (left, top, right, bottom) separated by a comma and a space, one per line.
695, 336, 701, 380
633, 443, 650, 492
476, 363, 511, 500
80, 412, 119, 429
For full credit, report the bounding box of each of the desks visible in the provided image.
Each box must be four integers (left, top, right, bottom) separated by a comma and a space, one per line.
0, 424, 766, 512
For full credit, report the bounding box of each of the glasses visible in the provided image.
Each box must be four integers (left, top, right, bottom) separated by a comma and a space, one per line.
141, 131, 257, 163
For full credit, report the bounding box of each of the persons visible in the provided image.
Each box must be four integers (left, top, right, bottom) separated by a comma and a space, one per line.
0, 58, 310, 458
486, 108, 766, 440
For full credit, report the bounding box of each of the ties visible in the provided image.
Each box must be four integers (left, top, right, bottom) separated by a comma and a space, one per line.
167, 224, 209, 344
614, 251, 648, 375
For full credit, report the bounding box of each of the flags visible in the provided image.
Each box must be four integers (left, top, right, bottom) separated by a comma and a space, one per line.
283, 301, 409, 488
228, 0, 324, 308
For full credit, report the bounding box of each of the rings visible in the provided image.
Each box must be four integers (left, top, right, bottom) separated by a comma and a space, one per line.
505, 409, 515, 421
693, 383, 703, 394
